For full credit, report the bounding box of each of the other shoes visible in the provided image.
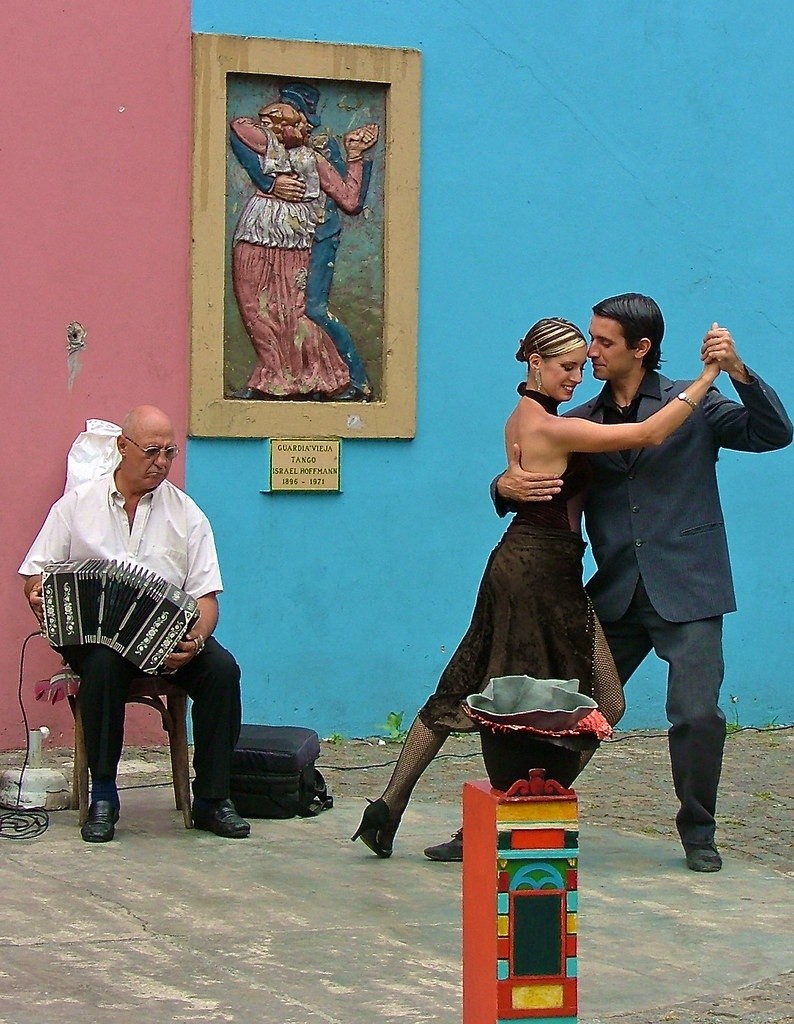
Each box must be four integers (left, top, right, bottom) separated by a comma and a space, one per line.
685, 844, 722, 872
424, 827, 463, 860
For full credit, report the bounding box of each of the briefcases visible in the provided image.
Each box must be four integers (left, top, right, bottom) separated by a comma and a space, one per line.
231, 724, 333, 819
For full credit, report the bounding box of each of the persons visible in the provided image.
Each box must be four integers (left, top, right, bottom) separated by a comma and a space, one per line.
19, 404, 253, 840
424, 292, 794, 872
352, 316, 721, 858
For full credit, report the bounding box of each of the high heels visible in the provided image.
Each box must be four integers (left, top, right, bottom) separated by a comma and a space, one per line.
350, 798, 393, 858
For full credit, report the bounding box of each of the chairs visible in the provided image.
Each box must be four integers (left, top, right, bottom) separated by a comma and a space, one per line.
33, 657, 194, 831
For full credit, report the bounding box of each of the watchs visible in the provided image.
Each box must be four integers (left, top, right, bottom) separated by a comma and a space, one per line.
678, 393, 697, 410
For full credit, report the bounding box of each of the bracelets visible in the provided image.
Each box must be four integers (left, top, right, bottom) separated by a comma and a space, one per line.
192, 635, 205, 655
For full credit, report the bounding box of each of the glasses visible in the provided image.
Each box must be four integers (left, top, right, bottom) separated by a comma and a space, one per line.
126, 436, 180, 460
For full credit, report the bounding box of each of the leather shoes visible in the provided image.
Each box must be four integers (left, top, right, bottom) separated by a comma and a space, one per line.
191, 798, 250, 838
81, 798, 120, 843
